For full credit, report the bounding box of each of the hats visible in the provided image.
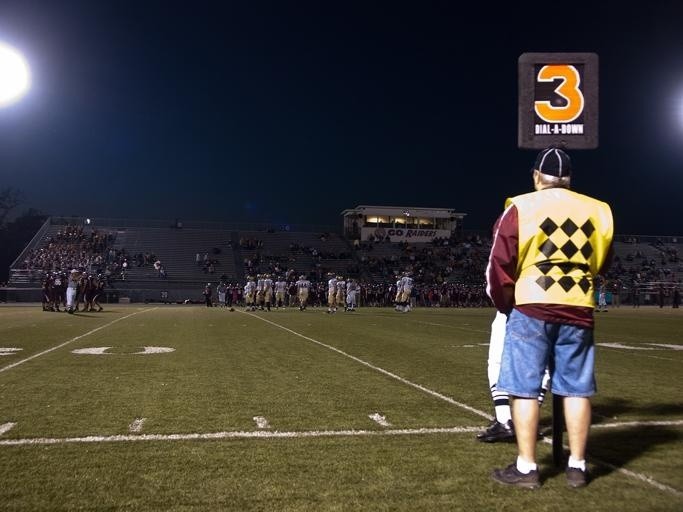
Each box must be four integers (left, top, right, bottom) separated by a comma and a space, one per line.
530, 145, 580, 177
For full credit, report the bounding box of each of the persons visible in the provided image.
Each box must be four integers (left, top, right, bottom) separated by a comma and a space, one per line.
596, 234, 682, 313
22, 213, 492, 313
475, 312, 554, 443
482, 143, 617, 486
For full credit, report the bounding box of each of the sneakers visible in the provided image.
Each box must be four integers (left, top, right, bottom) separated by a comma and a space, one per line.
566, 466, 590, 488
477, 419, 516, 443
491, 463, 541, 488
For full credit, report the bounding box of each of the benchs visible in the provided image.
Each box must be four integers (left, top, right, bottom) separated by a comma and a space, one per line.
8, 214, 495, 289
604, 235, 683, 295
